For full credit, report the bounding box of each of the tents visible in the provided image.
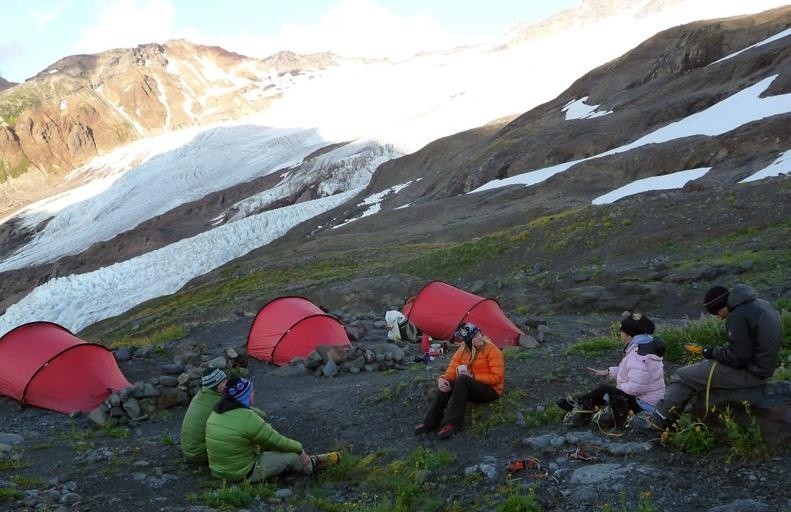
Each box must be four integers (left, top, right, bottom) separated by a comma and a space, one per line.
0, 320, 134, 416
401, 279, 525, 351
243, 295, 353, 368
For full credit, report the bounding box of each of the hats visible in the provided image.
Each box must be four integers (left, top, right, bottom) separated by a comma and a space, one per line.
622, 314, 654, 336
201, 368, 226, 389
460, 322, 480, 349
226, 377, 253, 401
704, 286, 729, 312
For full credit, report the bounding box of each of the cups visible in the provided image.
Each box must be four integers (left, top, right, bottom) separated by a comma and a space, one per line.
431, 343, 442, 353
456, 365, 468, 376
420, 335, 430, 352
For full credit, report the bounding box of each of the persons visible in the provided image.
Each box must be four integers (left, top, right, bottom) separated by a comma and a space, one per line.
180, 367, 268, 465
556, 310, 668, 425
413, 322, 505, 439
205, 376, 343, 486
632, 282, 781, 444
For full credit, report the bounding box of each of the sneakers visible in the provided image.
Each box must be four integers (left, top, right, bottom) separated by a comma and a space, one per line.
416, 423, 440, 432
437, 424, 457, 438
311, 452, 341, 472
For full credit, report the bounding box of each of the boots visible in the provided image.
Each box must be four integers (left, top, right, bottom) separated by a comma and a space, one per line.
632, 399, 681, 437
558, 389, 605, 410
593, 398, 628, 426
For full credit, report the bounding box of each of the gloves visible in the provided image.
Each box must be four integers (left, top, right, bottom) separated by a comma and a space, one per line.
702, 347, 713, 358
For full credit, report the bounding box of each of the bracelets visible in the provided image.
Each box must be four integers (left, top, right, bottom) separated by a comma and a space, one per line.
608, 369, 610, 376
